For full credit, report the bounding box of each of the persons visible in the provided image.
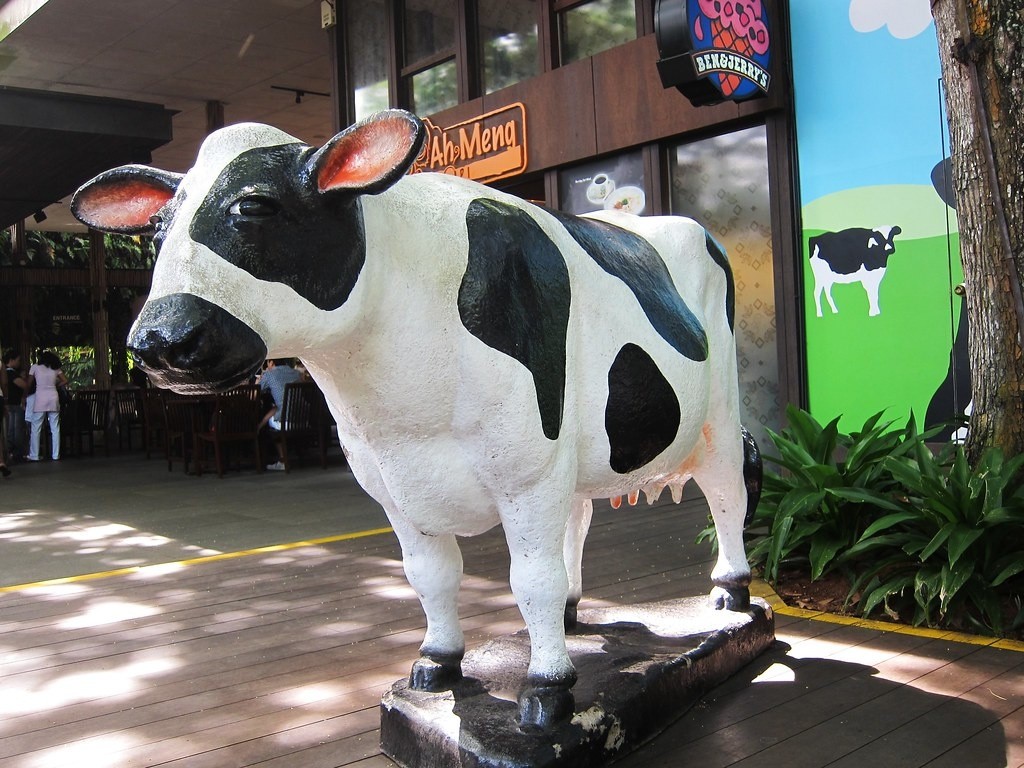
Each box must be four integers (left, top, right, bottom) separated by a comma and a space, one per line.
0, 348, 306, 478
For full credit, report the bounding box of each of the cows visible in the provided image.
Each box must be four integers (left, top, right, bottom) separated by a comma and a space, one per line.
69, 109, 752, 729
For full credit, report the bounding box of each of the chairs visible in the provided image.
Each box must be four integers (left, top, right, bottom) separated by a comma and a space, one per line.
61, 383, 328, 480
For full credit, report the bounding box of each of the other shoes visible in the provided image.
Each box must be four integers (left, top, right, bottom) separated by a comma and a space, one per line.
0, 465, 11, 477
27, 455, 39, 460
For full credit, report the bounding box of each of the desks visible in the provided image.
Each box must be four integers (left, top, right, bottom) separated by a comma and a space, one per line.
175, 397, 244, 463
78, 387, 149, 454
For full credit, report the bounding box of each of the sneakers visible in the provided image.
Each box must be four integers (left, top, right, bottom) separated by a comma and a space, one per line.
267, 460, 285, 471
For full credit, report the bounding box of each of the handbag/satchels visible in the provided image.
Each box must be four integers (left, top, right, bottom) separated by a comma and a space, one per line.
25, 392, 46, 423
58, 383, 69, 404
